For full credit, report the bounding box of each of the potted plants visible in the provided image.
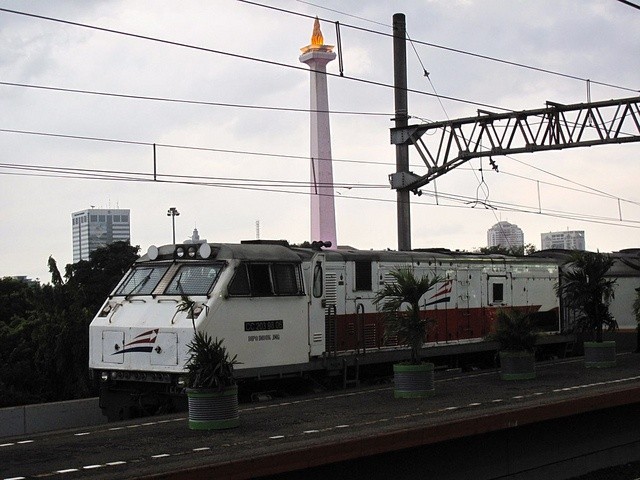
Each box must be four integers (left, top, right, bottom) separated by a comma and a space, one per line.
370, 262, 450, 399
184, 330, 244, 431
480, 305, 544, 381
553, 248, 628, 370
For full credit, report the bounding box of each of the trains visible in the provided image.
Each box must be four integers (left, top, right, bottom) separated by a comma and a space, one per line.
89, 240, 640, 423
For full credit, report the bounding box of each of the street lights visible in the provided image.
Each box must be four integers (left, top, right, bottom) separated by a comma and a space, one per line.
167, 207, 180, 244
